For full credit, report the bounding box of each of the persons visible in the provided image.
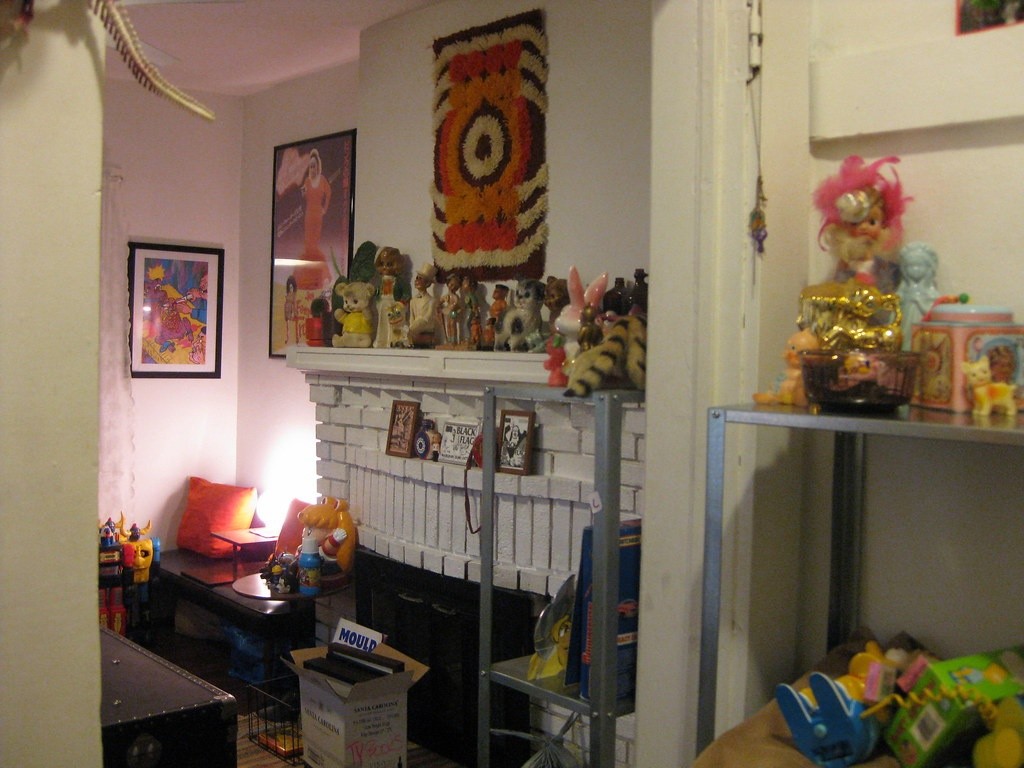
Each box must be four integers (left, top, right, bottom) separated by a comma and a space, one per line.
459, 276, 483, 343
408, 262, 439, 334
435, 273, 463, 344
284, 277, 298, 345
816, 156, 905, 295
372, 247, 412, 348
890, 241, 942, 352
482, 284, 510, 350
300, 148, 332, 261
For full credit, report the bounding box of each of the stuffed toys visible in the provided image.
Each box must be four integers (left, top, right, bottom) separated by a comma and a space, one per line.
332, 282, 375, 346
563, 314, 647, 398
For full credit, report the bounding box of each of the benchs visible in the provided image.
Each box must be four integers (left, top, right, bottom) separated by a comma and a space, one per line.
154, 549, 319, 698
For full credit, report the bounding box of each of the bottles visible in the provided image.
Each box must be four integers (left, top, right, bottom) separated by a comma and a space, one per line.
298, 538, 322, 596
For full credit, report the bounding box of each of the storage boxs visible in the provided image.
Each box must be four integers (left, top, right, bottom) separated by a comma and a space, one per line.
881, 642, 1024, 768
175, 599, 224, 642
244, 620, 430, 768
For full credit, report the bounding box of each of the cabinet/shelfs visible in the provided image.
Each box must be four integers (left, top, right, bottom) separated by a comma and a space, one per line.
481, 382, 649, 768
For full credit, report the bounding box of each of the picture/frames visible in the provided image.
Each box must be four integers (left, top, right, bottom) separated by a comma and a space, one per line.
268, 127, 357, 357
436, 419, 480, 466
126, 242, 228, 378
497, 410, 536, 476
387, 400, 420, 459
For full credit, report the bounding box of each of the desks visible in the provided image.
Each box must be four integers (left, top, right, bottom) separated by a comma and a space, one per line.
209, 525, 281, 582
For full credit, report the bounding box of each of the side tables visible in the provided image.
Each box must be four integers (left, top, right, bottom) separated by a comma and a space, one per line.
233, 570, 371, 691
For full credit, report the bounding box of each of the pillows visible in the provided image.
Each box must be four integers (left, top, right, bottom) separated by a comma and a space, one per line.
276, 498, 314, 555
176, 475, 261, 558
